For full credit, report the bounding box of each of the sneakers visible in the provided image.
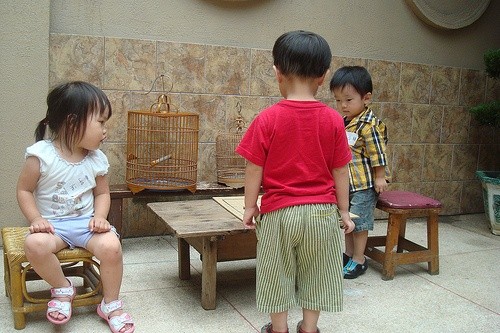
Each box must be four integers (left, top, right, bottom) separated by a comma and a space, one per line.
343, 256, 368, 279
343, 253, 352, 268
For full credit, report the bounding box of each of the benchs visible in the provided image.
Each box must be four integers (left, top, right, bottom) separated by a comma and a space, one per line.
106, 182, 263, 245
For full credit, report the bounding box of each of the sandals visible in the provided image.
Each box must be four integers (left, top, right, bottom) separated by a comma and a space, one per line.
47, 278, 76, 324
97, 297, 134, 333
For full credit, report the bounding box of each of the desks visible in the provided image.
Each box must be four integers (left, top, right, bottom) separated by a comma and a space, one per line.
146, 194, 360, 311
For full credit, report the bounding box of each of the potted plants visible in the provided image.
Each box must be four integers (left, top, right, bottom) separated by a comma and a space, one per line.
470, 48, 500, 236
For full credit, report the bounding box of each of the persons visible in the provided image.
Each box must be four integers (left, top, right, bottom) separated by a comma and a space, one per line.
330, 66, 393, 279
16, 81, 135, 333
236, 30, 355, 333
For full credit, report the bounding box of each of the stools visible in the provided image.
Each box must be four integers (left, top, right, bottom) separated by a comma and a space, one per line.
367, 192, 441, 280
2, 226, 109, 330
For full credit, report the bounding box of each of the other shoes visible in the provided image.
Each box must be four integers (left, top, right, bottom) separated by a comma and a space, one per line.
297, 320, 320, 333
261, 323, 289, 333
